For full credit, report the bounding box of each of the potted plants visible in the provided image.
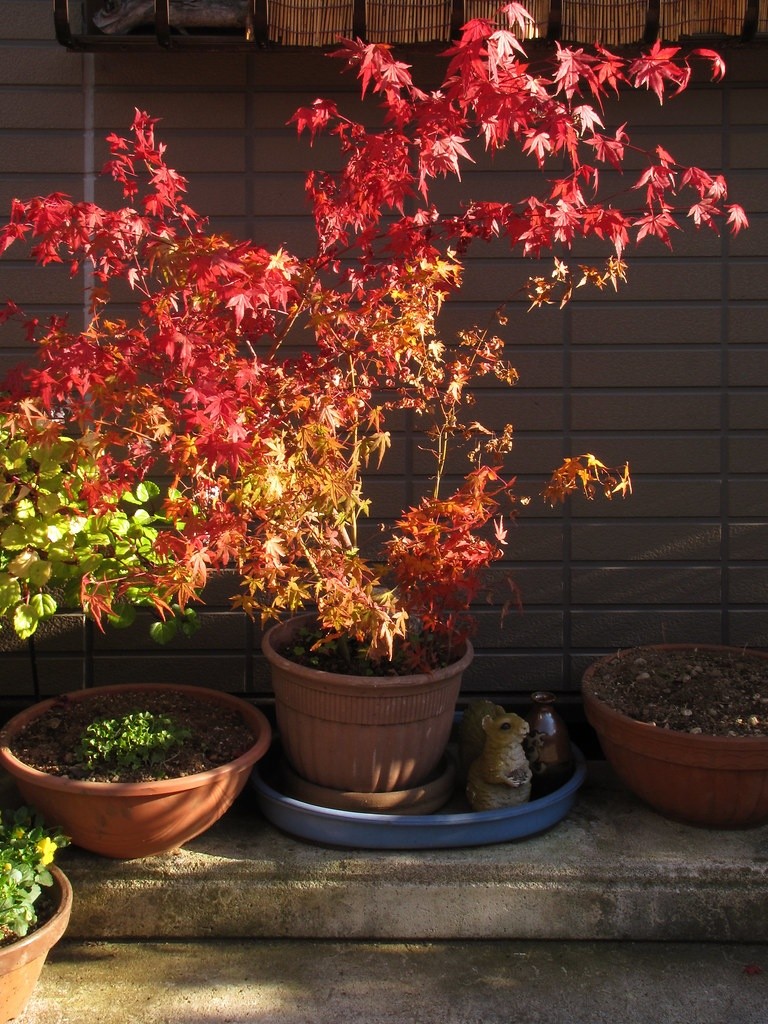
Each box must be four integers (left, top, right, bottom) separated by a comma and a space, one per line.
0, 683, 269, 855
0, 808, 73, 1021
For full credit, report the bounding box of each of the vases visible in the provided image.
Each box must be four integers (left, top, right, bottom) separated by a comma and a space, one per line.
262, 610, 476, 790
582, 646, 768, 829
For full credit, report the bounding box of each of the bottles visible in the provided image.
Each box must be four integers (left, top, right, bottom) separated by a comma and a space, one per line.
520, 692, 574, 788
